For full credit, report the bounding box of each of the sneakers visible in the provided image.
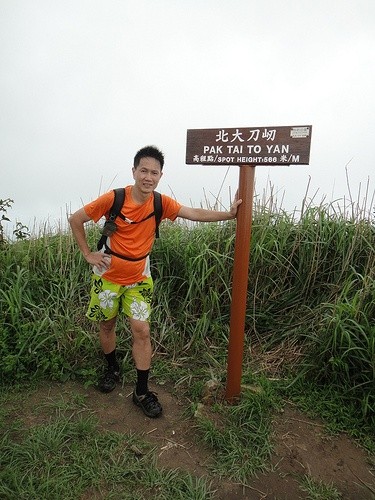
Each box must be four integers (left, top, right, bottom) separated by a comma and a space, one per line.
133, 386, 165, 418
98, 370, 120, 392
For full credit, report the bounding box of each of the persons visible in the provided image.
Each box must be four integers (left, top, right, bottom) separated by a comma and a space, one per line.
69, 144, 243, 418
